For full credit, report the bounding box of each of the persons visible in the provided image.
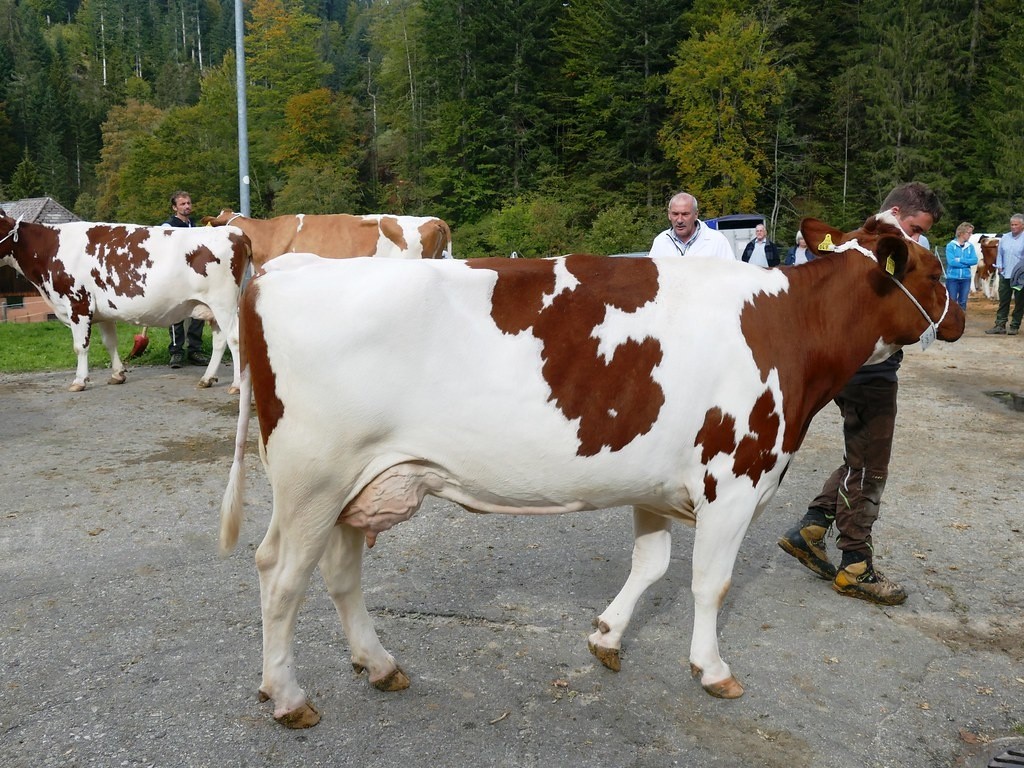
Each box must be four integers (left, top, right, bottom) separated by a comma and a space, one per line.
742, 224, 780, 268
647, 193, 735, 258
984, 213, 1024, 335
785, 230, 815, 266
159, 190, 212, 369
945, 222, 978, 311
777, 180, 941, 606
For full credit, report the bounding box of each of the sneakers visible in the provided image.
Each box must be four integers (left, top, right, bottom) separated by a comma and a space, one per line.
170, 353, 183, 368
187, 352, 209, 366
985, 324, 1006, 335
779, 521, 838, 580
1008, 327, 1018, 334
833, 559, 906, 606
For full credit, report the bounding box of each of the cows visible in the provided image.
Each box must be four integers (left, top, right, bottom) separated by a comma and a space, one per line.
980, 239, 998, 303
206, 210, 452, 385
222, 218, 965, 732
3, 214, 261, 394
